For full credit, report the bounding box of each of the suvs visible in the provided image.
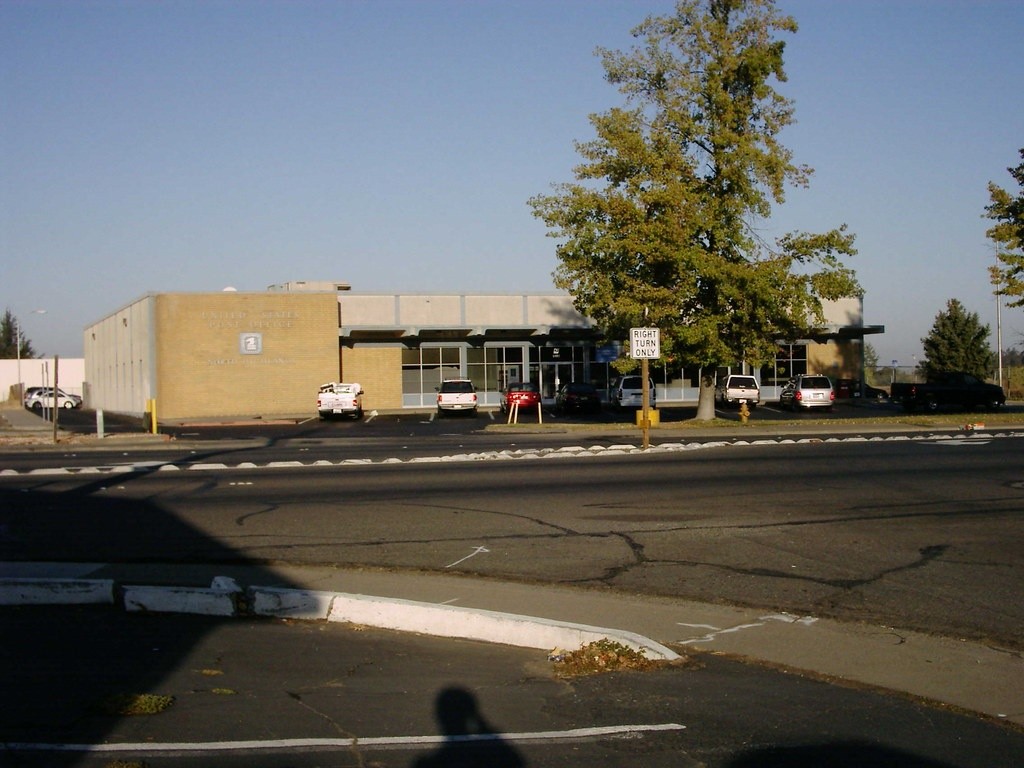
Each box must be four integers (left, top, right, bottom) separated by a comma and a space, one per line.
24, 387, 82, 404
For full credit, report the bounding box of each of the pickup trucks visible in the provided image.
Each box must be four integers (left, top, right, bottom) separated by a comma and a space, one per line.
317, 381, 365, 418
889, 370, 1005, 412
434, 378, 478, 413
715, 376, 761, 406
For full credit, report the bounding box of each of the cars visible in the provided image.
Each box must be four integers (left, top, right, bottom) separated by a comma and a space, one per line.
27, 390, 82, 409
500, 383, 543, 412
833, 379, 890, 403
554, 381, 602, 414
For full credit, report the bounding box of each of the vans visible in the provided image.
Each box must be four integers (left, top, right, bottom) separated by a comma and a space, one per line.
780, 375, 835, 412
611, 375, 656, 411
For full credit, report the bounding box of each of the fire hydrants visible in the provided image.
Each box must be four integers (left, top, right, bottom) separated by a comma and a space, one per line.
738, 402, 750, 425
